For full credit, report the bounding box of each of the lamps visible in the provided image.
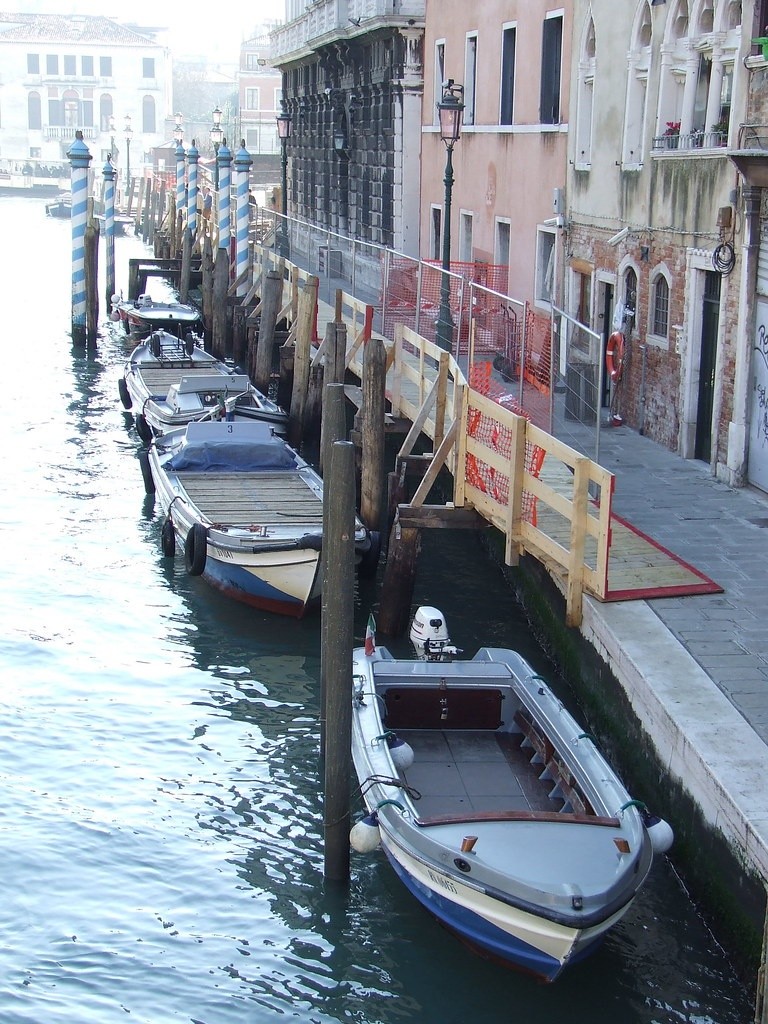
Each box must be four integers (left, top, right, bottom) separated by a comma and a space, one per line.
333, 123, 346, 153
348, 16, 369, 26
257, 59, 269, 65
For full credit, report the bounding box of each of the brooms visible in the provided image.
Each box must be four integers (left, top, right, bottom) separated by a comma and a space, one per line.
612, 380, 623, 427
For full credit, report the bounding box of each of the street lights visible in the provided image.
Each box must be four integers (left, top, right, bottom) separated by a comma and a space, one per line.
123, 114, 133, 197
209, 106, 224, 192
108, 116, 115, 163
172, 112, 185, 184
275, 108, 295, 281
427, 76, 468, 389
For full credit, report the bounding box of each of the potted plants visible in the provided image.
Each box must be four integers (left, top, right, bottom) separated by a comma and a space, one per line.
710, 123, 728, 147
688, 129, 703, 147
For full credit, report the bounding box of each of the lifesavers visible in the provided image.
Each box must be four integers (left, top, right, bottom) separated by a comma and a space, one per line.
234, 365, 247, 375
162, 520, 175, 557
605, 330, 624, 386
136, 414, 153, 442
184, 524, 207, 576
154, 334, 161, 357
360, 531, 381, 570
493, 356, 510, 382
119, 379, 132, 409
125, 318, 130, 334
186, 333, 193, 355
140, 453, 155, 493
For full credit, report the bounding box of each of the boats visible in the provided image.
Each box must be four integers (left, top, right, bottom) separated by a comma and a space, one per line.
146, 419, 372, 621
350, 604, 676, 988
119, 326, 289, 445
92, 208, 134, 236
111, 293, 203, 343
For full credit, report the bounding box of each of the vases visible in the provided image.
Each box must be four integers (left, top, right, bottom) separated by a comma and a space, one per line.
666, 136, 678, 148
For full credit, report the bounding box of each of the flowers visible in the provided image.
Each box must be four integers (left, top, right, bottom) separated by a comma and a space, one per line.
661, 118, 681, 136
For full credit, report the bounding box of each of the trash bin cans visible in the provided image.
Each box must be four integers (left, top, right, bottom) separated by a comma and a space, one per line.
565, 362, 594, 421
